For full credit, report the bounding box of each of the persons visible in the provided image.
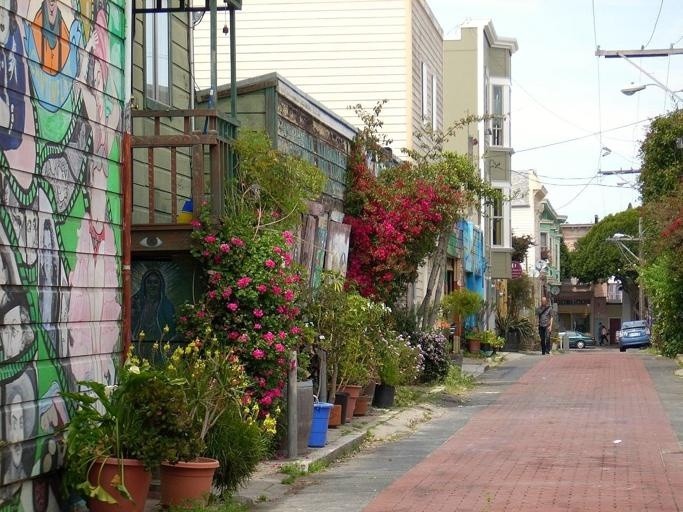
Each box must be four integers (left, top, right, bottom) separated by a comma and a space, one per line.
535, 295, 554, 355
597, 322, 608, 346
131, 267, 180, 367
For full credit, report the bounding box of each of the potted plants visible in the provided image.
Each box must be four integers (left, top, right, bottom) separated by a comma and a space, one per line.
467, 329, 506, 353
158, 339, 269, 512
314, 269, 419, 425
56, 364, 208, 512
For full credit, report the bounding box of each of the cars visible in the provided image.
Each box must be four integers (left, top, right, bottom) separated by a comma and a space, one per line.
619, 320, 651, 352
559, 330, 594, 348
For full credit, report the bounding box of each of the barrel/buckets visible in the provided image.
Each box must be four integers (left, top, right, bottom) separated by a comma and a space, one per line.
307, 402, 334, 448
